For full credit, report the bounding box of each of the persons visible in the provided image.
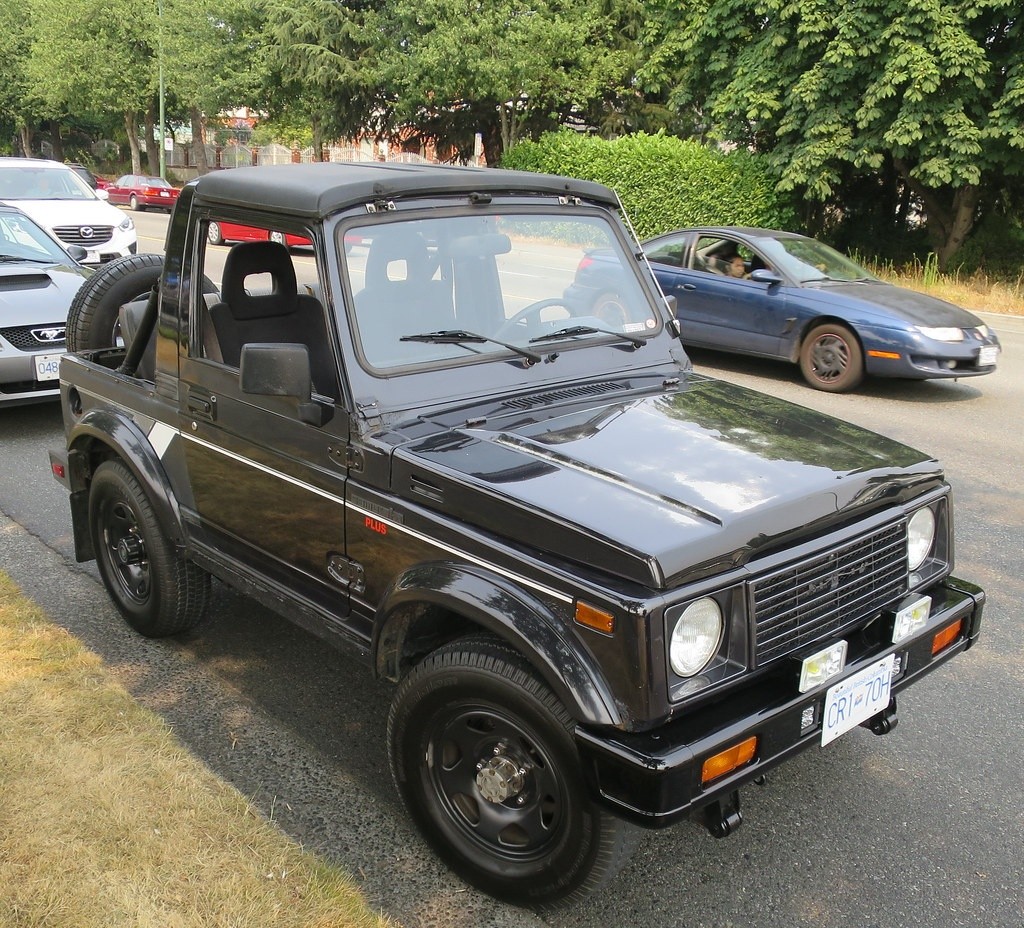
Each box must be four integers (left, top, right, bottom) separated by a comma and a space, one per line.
724, 254, 826, 280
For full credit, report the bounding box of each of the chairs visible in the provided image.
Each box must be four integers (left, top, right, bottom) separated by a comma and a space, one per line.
209, 240, 342, 404
354, 231, 454, 362
705, 254, 726, 275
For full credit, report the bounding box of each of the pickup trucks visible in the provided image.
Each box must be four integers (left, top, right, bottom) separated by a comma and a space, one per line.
45, 160, 989, 917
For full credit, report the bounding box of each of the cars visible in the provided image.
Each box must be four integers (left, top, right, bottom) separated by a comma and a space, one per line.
0, 203, 129, 410
63, 163, 111, 192
0, 156, 140, 270
105, 174, 181, 214
559, 225, 1004, 394
205, 219, 363, 255
